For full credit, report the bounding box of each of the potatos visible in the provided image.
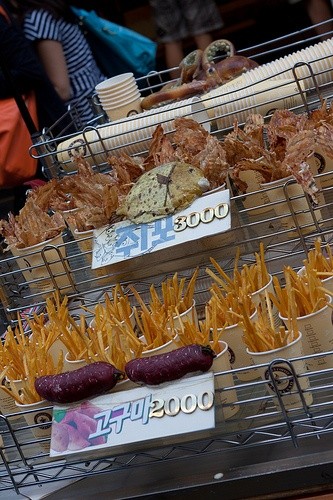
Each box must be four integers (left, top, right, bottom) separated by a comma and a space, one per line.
126, 343, 213, 386
34, 361, 126, 403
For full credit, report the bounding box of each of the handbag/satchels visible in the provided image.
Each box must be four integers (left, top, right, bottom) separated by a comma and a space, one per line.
77, 8, 157, 81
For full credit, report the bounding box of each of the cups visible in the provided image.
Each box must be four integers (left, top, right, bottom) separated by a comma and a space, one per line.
10, 36, 333, 291
0, 259, 333, 453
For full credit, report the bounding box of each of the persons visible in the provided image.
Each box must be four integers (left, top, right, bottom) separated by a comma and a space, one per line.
8, 0, 222, 129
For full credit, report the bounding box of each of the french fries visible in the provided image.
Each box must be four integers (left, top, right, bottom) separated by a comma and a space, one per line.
0, 238, 333, 402
